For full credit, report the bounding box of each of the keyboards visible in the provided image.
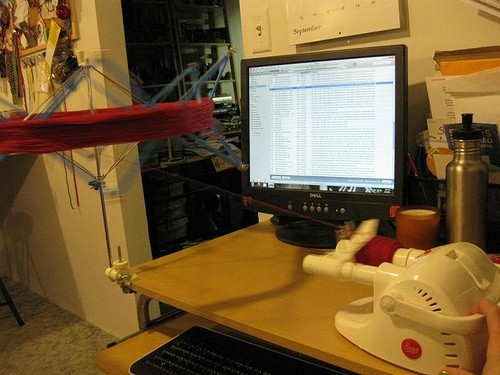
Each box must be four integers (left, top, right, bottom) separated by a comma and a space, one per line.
130, 326, 344, 375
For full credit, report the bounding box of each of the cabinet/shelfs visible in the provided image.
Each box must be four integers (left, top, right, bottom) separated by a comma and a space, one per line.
121, 0, 241, 125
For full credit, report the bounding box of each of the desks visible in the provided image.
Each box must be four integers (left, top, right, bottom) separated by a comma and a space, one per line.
142, 156, 211, 259
92, 220, 500, 375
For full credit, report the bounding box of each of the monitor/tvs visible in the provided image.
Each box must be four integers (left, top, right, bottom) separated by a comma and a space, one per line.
240, 44, 410, 249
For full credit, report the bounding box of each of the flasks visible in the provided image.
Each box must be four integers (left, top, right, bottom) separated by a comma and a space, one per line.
445, 113, 489, 254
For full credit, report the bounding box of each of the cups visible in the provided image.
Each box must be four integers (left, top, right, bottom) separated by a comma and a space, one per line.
394, 204, 440, 251
